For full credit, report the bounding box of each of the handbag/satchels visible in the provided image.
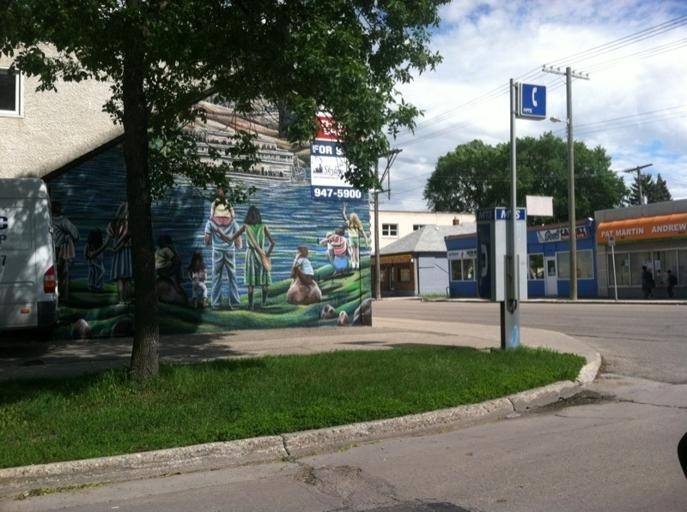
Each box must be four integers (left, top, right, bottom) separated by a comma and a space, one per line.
261, 252, 272, 272
331, 241, 341, 247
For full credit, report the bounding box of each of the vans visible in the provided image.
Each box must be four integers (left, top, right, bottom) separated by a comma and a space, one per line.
0, 176, 60, 334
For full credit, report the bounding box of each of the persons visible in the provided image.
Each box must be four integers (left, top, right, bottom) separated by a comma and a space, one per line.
288, 246, 315, 285
341, 202, 369, 271
209, 186, 236, 226
205, 217, 243, 310
211, 205, 277, 310
85, 199, 134, 306
314, 227, 351, 256
665, 269, 674, 299
82, 228, 109, 295
186, 247, 210, 307
640, 265, 654, 299
154, 230, 186, 286
50, 200, 81, 300
319, 231, 356, 279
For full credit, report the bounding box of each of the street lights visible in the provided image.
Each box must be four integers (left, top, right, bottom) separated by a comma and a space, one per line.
548, 116, 579, 302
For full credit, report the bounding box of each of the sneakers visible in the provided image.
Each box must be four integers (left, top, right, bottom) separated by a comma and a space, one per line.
351, 261, 360, 269
212, 300, 268, 310
